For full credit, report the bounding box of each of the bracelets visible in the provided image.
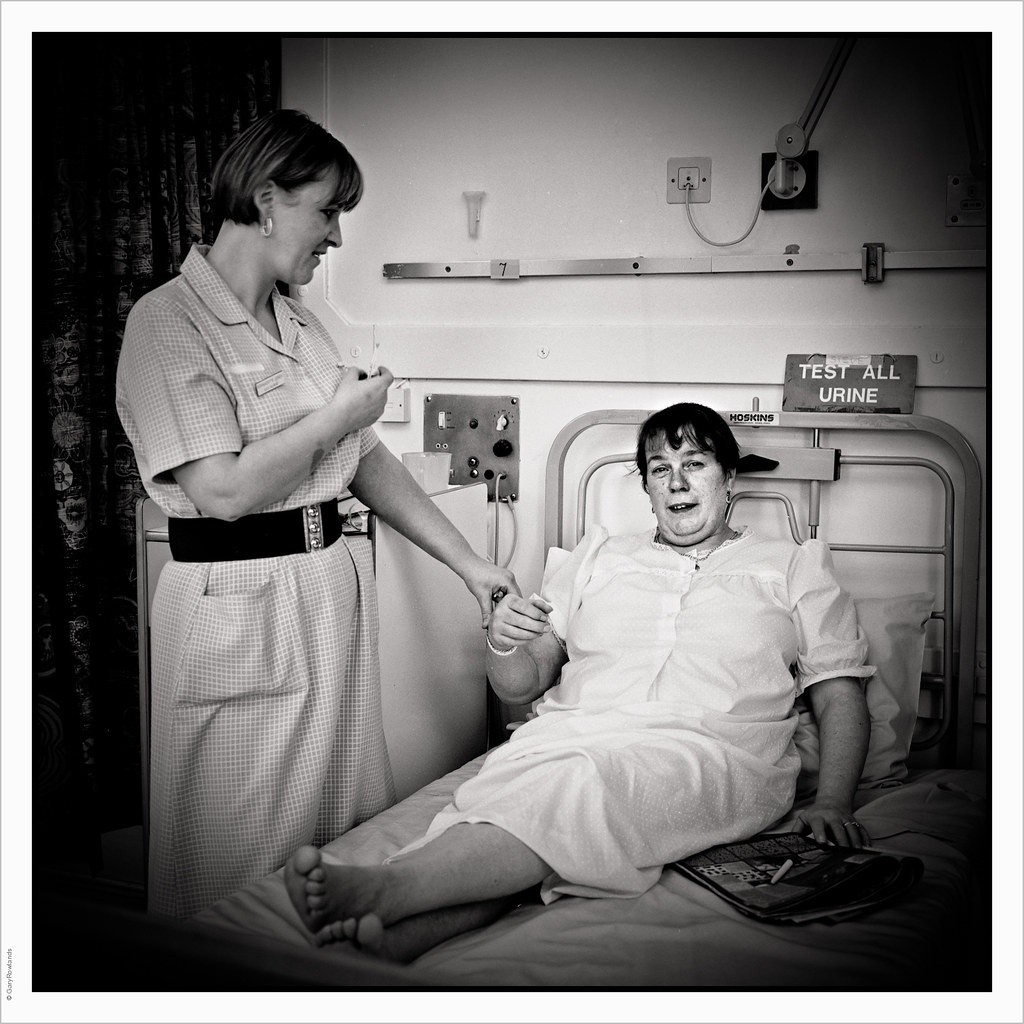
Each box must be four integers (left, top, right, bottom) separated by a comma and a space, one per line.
485, 630, 518, 656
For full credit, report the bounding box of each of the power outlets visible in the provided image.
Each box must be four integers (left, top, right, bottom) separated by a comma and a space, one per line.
667, 157, 712, 204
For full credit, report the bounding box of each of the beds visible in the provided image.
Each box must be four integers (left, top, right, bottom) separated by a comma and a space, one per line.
32, 411, 985, 987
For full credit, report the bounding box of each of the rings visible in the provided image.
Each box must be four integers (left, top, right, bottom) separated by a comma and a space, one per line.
842, 820, 850, 826
852, 822, 861, 827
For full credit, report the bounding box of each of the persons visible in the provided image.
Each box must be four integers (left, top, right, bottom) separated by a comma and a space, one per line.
283, 404, 877, 966
116, 110, 523, 923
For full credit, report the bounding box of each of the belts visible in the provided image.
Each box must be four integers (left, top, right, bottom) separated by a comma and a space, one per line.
164, 498, 346, 563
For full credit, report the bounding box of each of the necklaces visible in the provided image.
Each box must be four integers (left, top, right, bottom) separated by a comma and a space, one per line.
657, 531, 739, 561
